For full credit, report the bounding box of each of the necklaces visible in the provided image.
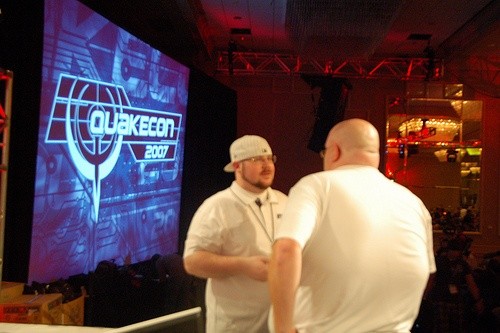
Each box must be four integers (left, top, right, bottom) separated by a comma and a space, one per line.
249, 204, 274, 243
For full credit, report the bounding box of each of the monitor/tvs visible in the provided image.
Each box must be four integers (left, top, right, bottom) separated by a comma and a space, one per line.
303, 73, 351, 156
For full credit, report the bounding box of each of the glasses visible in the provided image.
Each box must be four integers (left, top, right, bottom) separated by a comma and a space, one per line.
320, 146, 332, 158
247, 156, 276, 163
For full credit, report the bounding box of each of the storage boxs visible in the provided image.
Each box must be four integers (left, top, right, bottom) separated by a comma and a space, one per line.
0, 281, 90, 326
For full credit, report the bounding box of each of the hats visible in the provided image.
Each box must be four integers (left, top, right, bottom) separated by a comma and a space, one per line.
224, 135, 272, 172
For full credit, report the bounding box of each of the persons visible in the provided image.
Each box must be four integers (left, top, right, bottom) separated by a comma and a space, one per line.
267, 119, 437, 333
80, 254, 193, 327
427, 240, 485, 333
183, 135, 288, 333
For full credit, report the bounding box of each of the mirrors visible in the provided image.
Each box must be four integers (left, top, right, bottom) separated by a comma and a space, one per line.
383, 96, 484, 235
